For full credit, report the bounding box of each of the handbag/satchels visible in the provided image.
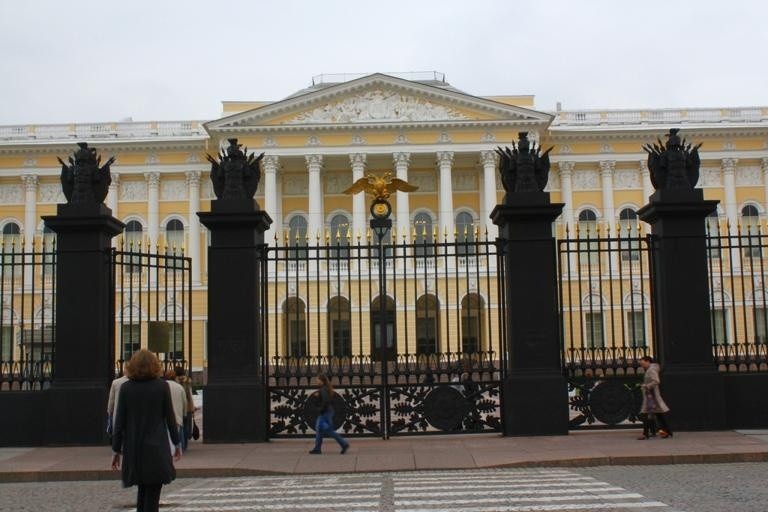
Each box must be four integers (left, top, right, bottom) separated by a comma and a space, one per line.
192, 424, 199, 440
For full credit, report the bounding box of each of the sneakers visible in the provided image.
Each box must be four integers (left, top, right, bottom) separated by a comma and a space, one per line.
341, 445, 349, 453
310, 450, 320, 454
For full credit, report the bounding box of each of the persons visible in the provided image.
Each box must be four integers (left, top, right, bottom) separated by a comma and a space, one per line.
106, 362, 195, 456
307, 373, 350, 455
635, 356, 674, 440
110, 348, 183, 511
648, 413, 657, 437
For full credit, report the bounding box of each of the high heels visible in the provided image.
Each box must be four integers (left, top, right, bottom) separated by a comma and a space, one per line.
662, 433, 672, 438
638, 435, 649, 440
